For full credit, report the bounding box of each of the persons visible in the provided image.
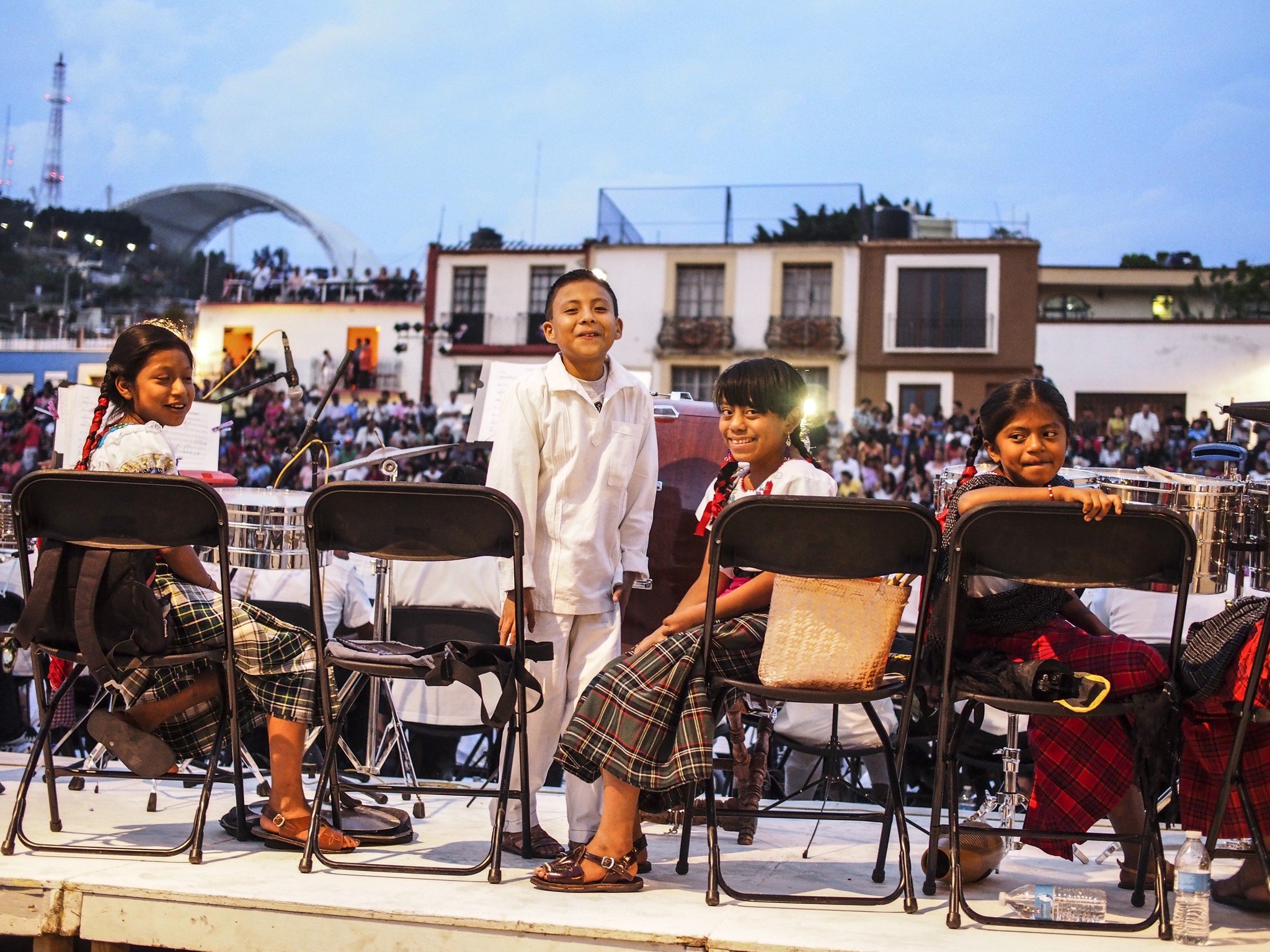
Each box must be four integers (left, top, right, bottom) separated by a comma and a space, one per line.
484, 267, 659, 862
922, 376, 1215, 893
531, 356, 844, 894
38, 323, 362, 854
0, 247, 1270, 495
0, 551, 1269, 824
1176, 593, 1269, 916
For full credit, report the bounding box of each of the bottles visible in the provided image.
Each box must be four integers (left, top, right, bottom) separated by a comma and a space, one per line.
1173, 832, 1211, 944
922, 820, 1003, 885
999, 884, 1107, 925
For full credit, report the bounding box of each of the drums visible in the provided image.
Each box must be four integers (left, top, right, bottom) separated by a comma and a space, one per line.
1076, 467, 1238, 595
940, 463, 1101, 504
192, 486, 334, 570
0, 493, 33, 550
1248, 480, 1270, 593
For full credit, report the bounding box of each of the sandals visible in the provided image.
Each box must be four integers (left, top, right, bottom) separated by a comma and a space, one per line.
498, 825, 565, 862
528, 843, 647, 894
631, 834, 653, 874
1206, 874, 1270, 912
1115, 856, 1217, 892
85, 705, 177, 779
249, 804, 360, 853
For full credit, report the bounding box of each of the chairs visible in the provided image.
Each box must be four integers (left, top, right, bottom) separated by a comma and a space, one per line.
297, 480, 537, 884
1183, 598, 1269, 907
673, 498, 944, 915
1, 470, 254, 867
920, 500, 1197, 940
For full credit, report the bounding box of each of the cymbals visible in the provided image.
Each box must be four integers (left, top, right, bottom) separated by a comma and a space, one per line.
312, 430, 460, 475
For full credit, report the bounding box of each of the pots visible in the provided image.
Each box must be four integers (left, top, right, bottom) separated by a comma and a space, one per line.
932, 463, 1270, 596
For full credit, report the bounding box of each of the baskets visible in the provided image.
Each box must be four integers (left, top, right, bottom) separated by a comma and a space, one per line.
757, 569, 913, 695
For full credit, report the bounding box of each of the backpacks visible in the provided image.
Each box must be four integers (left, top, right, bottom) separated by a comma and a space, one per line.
9, 533, 172, 694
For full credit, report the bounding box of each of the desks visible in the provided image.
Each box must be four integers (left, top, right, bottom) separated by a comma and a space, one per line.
220, 277, 405, 304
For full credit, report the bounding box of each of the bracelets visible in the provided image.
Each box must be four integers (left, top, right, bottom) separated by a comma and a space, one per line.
1048, 485, 1057, 502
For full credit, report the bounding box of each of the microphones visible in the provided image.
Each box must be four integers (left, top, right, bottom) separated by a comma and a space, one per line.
280, 330, 304, 400
